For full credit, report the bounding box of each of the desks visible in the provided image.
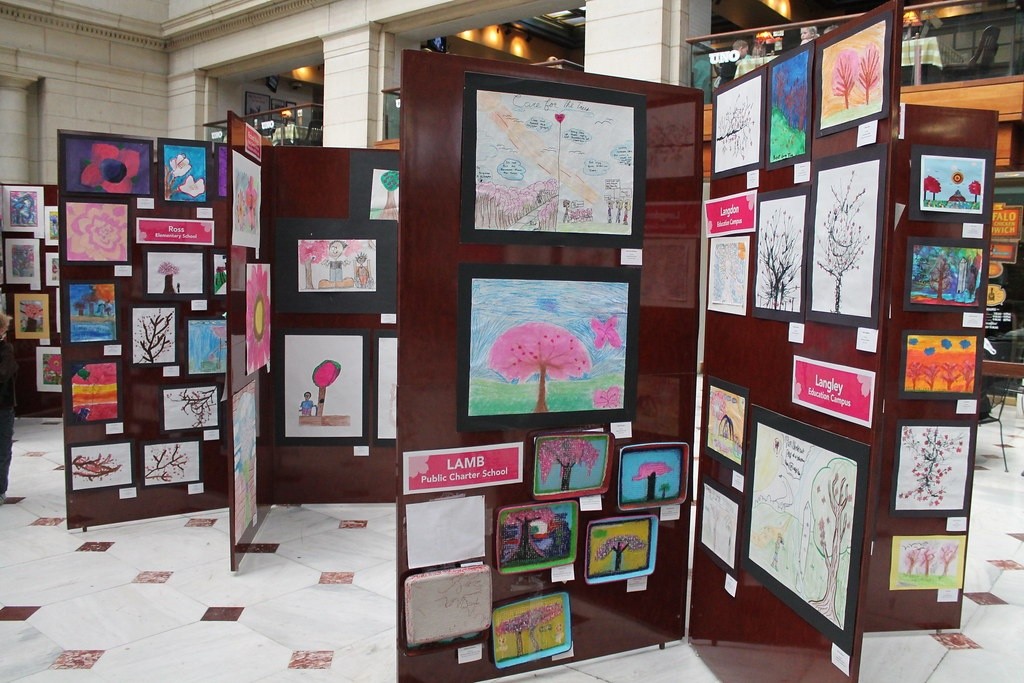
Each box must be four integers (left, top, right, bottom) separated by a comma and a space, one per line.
901, 36, 964, 85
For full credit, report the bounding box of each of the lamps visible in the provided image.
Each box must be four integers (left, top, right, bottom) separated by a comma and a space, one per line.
525, 35, 532, 43
504, 27, 511, 36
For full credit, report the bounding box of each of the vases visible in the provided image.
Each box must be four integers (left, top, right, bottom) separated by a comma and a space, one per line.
905, 26, 912, 40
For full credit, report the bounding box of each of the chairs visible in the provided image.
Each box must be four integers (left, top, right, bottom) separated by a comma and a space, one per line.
940, 25, 1002, 80
977, 373, 1014, 472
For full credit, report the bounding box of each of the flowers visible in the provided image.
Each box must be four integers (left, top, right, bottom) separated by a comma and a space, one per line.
903, 12, 923, 27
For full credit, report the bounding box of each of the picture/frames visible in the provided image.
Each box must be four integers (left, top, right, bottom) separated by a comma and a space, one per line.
271, 98, 285, 123
889, 418, 972, 518
897, 330, 981, 401
907, 144, 994, 224
742, 402, 872, 657
902, 235, 986, 313
285, 101, 297, 120
705, 374, 750, 475
245, 91, 270, 128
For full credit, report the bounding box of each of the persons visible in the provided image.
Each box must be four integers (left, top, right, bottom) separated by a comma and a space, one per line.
800, 26, 820, 45
0, 312, 19, 505
718, 40, 748, 86
824, 25, 839, 34
